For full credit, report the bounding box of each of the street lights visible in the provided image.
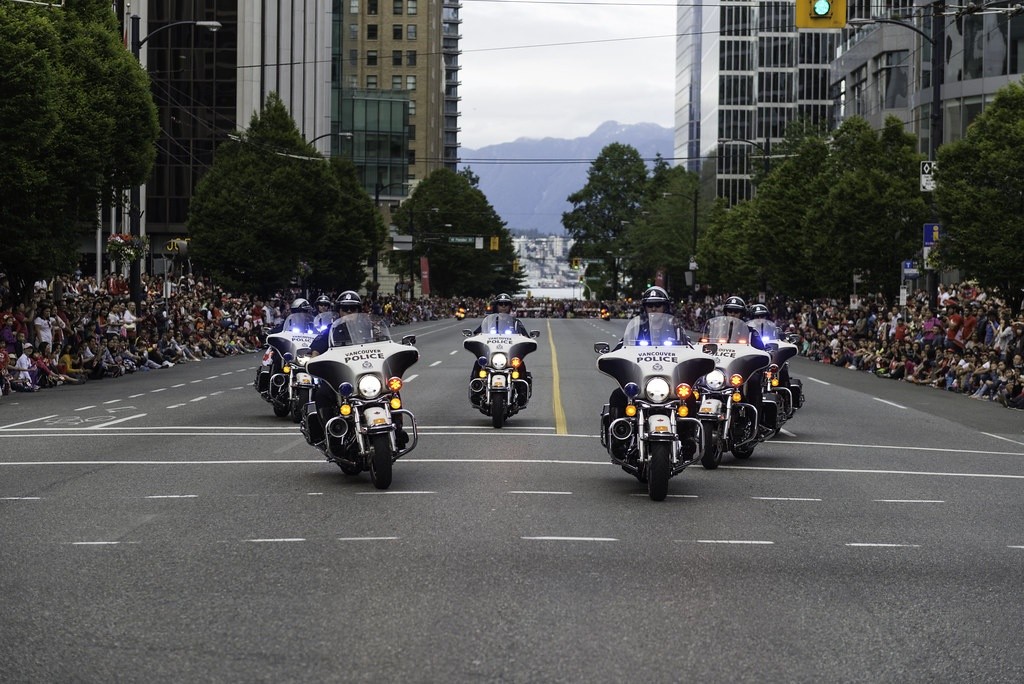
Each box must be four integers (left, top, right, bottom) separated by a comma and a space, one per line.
661, 190, 699, 302
719, 136, 771, 172
847, 0, 946, 315
306, 131, 354, 147
372, 181, 413, 301
130, 13, 222, 337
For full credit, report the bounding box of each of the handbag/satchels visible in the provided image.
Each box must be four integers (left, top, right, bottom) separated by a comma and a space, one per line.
1012, 379, 1022, 398
123, 310, 135, 331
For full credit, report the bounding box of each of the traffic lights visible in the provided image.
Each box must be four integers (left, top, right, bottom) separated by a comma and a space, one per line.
580, 276, 583, 285
795, 0, 847, 29
573, 259, 579, 269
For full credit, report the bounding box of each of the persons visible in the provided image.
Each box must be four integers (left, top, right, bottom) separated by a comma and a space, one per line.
265, 298, 313, 404
0, 264, 1024, 409
308, 290, 410, 452
469, 293, 530, 413
703, 296, 767, 439
608, 285, 701, 464
745, 304, 798, 414
312, 294, 331, 320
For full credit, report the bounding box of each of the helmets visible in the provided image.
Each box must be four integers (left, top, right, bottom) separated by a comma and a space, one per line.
315, 295, 330, 305
335, 290, 362, 305
724, 296, 747, 309
291, 298, 313, 308
496, 293, 512, 302
752, 304, 770, 315
643, 286, 669, 302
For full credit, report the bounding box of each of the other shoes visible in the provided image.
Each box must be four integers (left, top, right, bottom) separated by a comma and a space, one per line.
903, 377, 1009, 407
395, 429, 410, 443
848, 365, 857, 370
34, 379, 86, 390
240, 349, 262, 354
189, 358, 201, 362
205, 355, 214, 360
158, 360, 175, 369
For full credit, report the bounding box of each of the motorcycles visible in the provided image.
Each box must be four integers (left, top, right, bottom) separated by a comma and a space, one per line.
745, 318, 805, 438
461, 313, 537, 432
254, 313, 323, 425
593, 312, 715, 502
695, 317, 778, 473
299, 313, 421, 491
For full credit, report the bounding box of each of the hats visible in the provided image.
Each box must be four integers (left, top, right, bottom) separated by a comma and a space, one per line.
224, 312, 232, 318
24, 343, 33, 348
9, 354, 17, 359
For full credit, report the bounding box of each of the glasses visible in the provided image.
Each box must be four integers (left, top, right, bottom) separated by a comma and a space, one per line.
339, 305, 359, 313
319, 303, 329, 307
754, 315, 766, 318
725, 309, 741, 314
645, 302, 665, 308
498, 302, 509, 306
